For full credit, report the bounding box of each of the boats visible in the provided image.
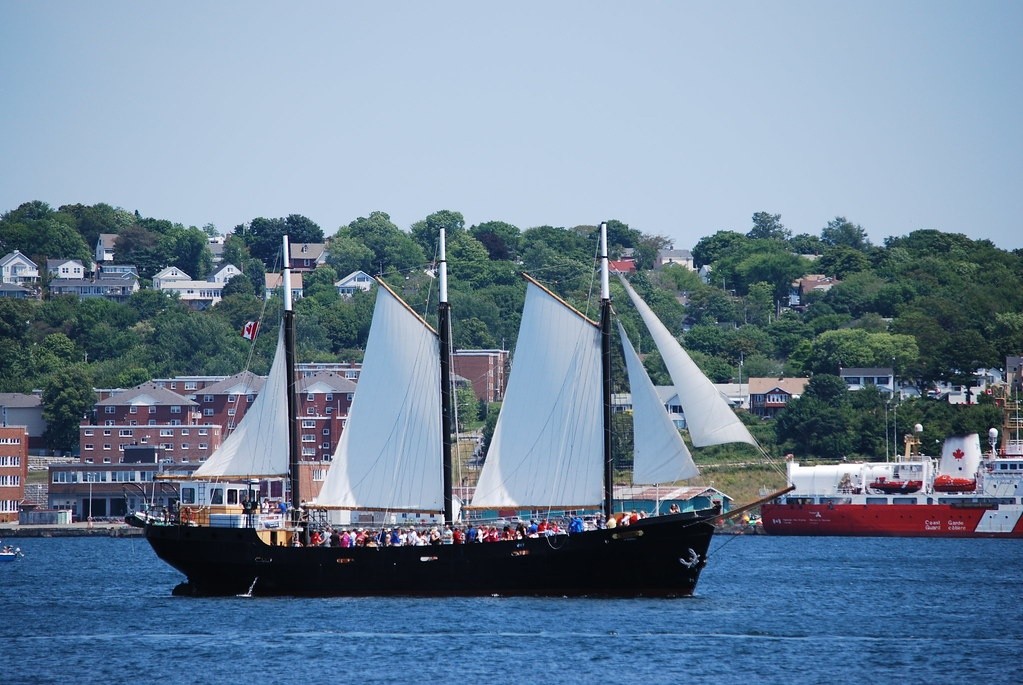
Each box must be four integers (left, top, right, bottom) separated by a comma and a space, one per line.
0, 547, 24, 561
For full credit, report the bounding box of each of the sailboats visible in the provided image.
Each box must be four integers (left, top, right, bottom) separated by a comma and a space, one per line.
119, 220, 797, 600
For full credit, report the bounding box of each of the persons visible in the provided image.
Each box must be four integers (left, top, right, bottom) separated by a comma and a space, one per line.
261, 497, 269, 515
278, 500, 286, 514
242, 495, 252, 527
269, 501, 278, 512
0, 545, 16, 554
296, 501, 681, 552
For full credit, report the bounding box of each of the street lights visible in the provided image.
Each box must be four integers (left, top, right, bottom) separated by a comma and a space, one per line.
85, 470, 98, 527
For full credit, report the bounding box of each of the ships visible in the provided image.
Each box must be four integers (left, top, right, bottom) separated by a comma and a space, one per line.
758, 424, 1023, 540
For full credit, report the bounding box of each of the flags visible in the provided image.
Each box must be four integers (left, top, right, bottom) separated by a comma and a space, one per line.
241, 320, 259, 341
